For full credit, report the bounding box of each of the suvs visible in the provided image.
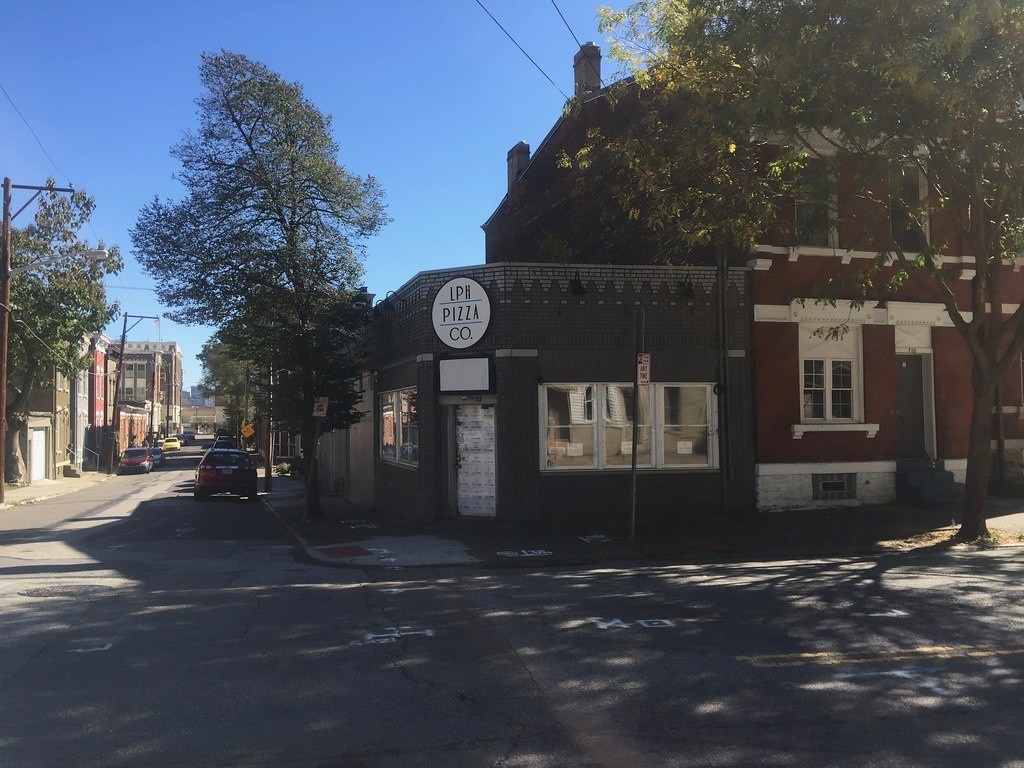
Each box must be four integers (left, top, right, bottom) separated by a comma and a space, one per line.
194, 447, 258, 503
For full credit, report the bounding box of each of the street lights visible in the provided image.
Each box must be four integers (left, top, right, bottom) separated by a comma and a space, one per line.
0, 249, 109, 505
107, 311, 159, 474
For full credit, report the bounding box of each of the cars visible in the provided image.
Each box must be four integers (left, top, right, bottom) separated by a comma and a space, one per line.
172, 430, 195, 446
150, 447, 166, 467
212, 428, 234, 448
117, 448, 154, 473
162, 437, 180, 451
